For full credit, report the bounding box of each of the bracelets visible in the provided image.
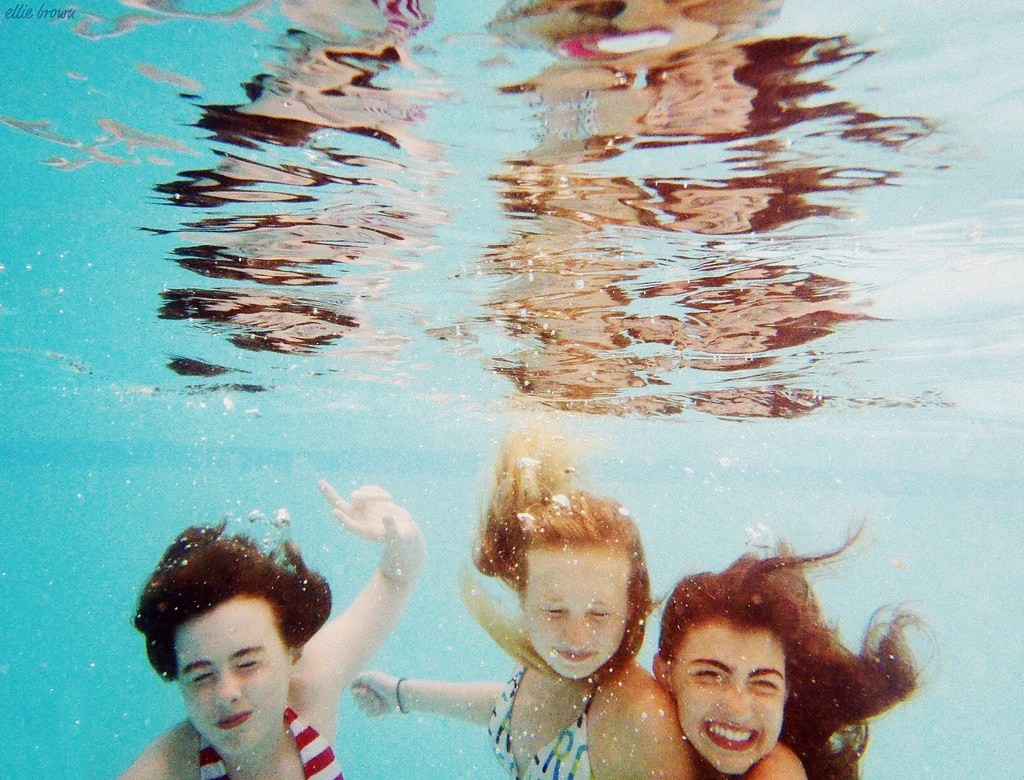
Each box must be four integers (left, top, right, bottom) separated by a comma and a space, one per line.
376, 563, 422, 586
396, 677, 411, 717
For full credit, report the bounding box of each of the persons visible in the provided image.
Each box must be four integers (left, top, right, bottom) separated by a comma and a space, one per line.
352, 421, 699, 780
118, 479, 430, 779
651, 512, 914, 780
